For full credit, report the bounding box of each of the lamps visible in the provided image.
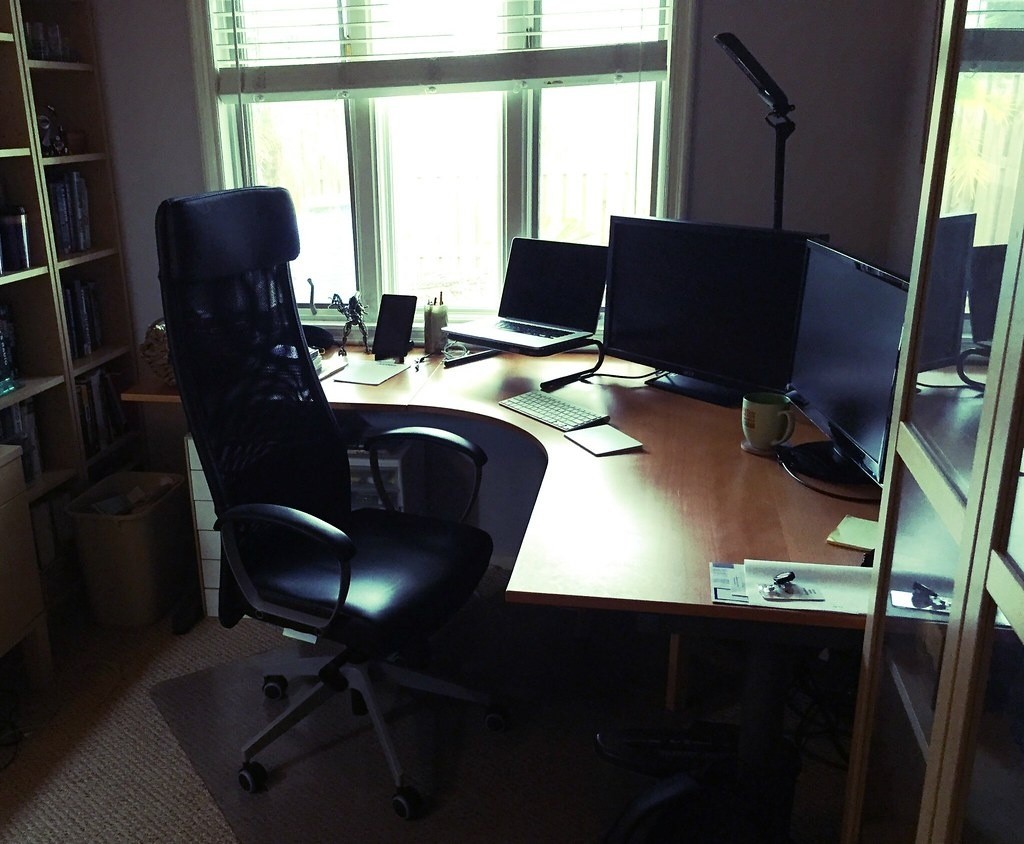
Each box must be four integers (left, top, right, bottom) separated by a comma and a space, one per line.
712, 32, 797, 231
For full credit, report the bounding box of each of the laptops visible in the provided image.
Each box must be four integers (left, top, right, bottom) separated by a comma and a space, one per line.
966, 245, 1008, 348
440, 238, 610, 351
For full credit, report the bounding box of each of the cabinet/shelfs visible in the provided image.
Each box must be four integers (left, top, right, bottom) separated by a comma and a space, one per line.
218, 567, 220, 568
0, 0, 89, 578
0, 444, 57, 686
14, 0, 138, 576
845, 99, 1024, 844
183, 431, 252, 619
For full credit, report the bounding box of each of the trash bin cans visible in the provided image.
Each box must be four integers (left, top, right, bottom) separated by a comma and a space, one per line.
63, 468, 187, 633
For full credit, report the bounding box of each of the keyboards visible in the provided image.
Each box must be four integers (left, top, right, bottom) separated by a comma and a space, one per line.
498, 389, 611, 432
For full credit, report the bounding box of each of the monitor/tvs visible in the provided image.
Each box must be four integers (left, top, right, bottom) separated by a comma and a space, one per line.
918, 213, 976, 373
601, 214, 829, 409
775, 237, 911, 484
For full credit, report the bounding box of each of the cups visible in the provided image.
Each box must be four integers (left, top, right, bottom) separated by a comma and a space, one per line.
0, 206, 32, 269
424, 304, 448, 354
742, 393, 796, 450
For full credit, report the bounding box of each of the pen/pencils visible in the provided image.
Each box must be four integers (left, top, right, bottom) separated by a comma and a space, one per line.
427, 291, 443, 312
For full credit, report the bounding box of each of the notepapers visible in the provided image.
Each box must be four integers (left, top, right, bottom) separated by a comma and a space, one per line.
827, 515, 878, 550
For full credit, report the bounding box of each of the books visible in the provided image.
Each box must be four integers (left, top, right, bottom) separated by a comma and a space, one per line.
63, 278, 105, 361
74, 368, 134, 459
1, 308, 19, 383
0, 201, 32, 274
45, 169, 93, 254
4, 399, 47, 485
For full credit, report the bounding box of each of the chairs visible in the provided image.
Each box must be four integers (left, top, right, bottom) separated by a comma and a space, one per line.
151, 184, 512, 821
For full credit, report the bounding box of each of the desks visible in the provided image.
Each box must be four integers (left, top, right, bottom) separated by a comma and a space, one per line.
116, 344, 880, 630
